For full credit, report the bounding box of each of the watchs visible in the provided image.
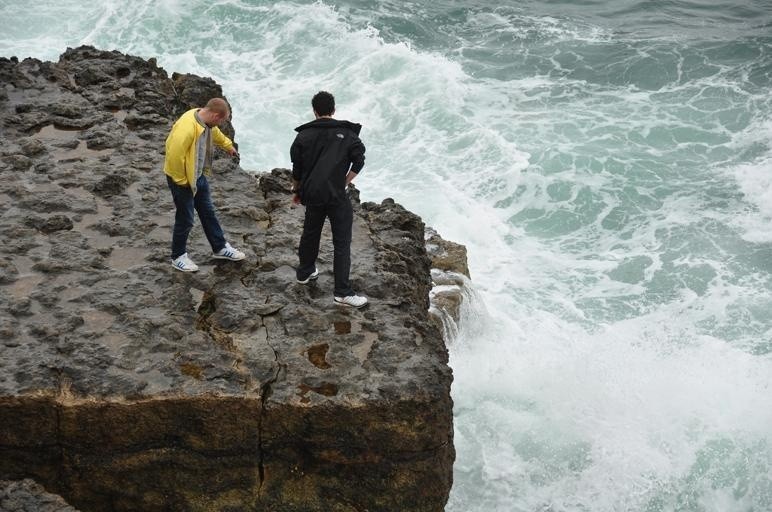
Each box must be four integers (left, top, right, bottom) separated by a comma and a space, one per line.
290, 186, 299, 194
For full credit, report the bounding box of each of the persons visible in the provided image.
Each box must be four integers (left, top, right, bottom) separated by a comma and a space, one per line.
163, 97, 245, 273
290, 91, 367, 309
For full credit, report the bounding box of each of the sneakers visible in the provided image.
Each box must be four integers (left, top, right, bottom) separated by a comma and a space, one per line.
171, 252, 198, 272
212, 242, 245, 261
296, 268, 318, 284
334, 294, 368, 308
